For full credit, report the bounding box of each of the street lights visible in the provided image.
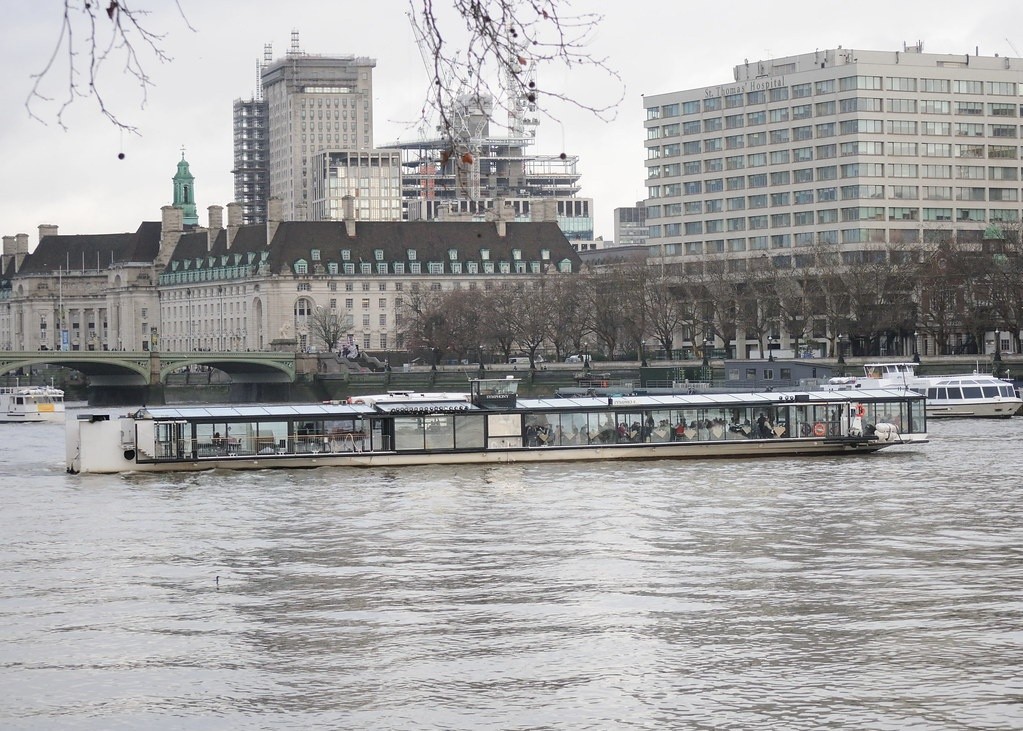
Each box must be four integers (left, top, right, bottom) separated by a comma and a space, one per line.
912, 331, 922, 363
584, 342, 590, 368
994, 327, 1001, 361
703, 337, 708, 366
837, 333, 845, 364
768, 336, 774, 362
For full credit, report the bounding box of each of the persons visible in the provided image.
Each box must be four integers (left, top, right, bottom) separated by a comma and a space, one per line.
524, 406, 917, 447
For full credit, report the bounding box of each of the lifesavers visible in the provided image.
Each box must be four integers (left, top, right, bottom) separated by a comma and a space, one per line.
814, 424, 826, 436
355, 399, 366, 405
855, 404, 865, 418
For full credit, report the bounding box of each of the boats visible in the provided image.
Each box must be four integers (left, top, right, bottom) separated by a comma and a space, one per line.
0, 383, 65, 417
66, 375, 928, 475
810, 363, 1023, 418
